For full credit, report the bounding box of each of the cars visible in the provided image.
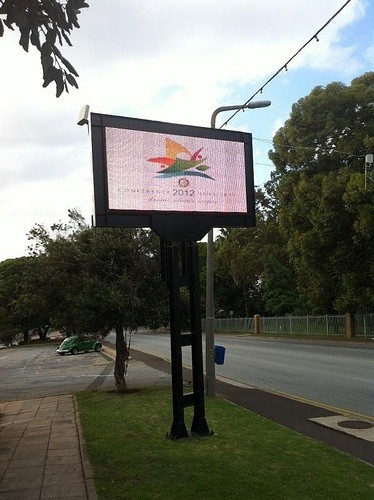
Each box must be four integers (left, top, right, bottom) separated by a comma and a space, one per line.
55, 335, 104, 355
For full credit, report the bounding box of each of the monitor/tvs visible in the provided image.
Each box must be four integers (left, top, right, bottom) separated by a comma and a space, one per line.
88, 111, 257, 228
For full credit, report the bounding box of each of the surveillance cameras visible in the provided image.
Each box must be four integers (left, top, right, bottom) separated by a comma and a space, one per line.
77, 104, 92, 126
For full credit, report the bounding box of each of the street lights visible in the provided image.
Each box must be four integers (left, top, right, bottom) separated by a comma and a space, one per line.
202, 99, 271, 397
